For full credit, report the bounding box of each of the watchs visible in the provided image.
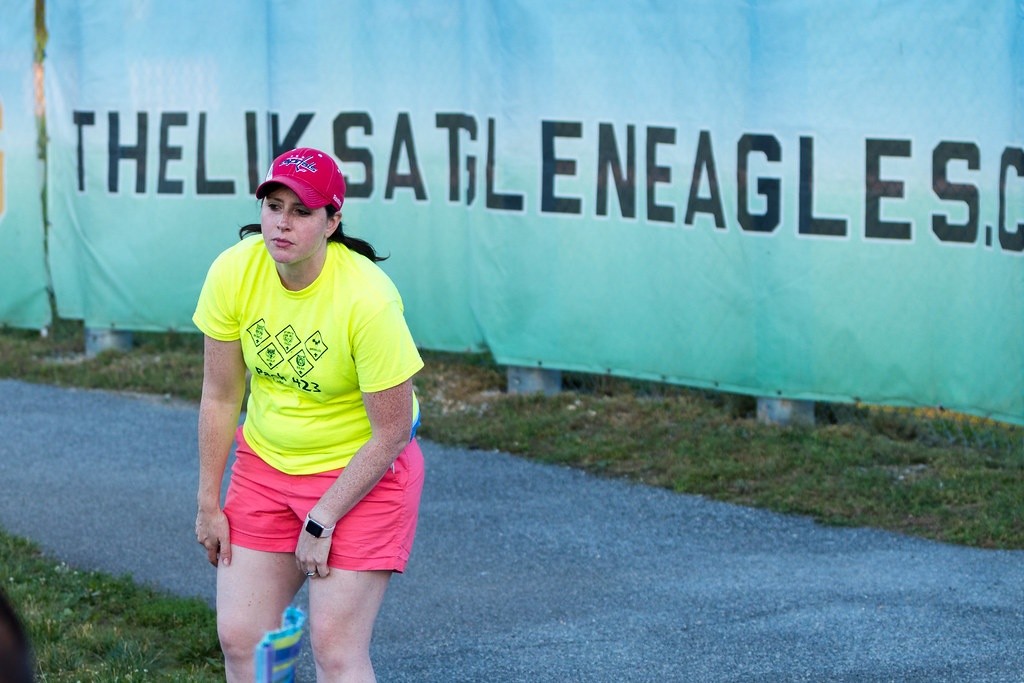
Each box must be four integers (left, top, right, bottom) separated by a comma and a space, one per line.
305, 512, 335, 538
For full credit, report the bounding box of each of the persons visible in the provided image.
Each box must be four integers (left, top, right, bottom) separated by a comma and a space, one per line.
191, 147, 425, 683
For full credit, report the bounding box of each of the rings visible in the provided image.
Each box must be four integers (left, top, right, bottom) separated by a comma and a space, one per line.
305, 572, 315, 576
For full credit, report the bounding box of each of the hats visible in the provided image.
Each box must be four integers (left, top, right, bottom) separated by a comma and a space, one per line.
255, 148, 346, 212
